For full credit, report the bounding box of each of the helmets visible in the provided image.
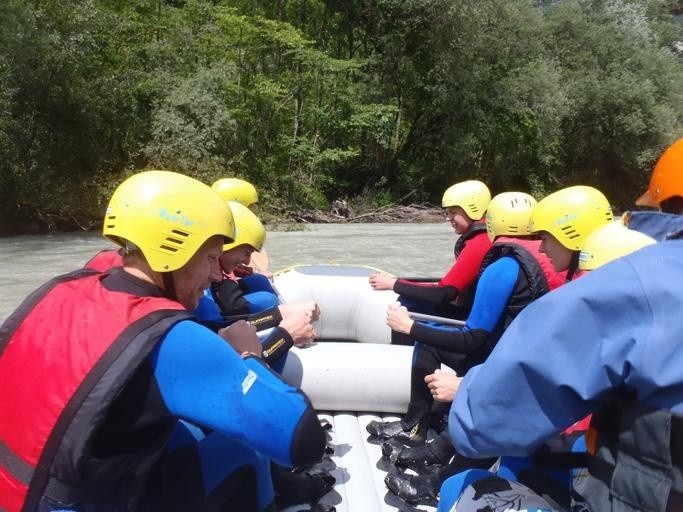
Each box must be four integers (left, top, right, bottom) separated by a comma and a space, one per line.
635, 137, 683, 208
103, 170, 266, 273
442, 180, 656, 269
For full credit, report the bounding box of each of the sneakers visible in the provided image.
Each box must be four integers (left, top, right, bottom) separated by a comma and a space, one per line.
366, 403, 458, 507
272, 462, 336, 509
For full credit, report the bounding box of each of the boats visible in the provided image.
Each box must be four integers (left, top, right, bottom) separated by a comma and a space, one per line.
266, 264, 415, 512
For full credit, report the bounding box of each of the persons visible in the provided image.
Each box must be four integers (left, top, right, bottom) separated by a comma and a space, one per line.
0, 170, 326, 511
367, 138, 683, 512
